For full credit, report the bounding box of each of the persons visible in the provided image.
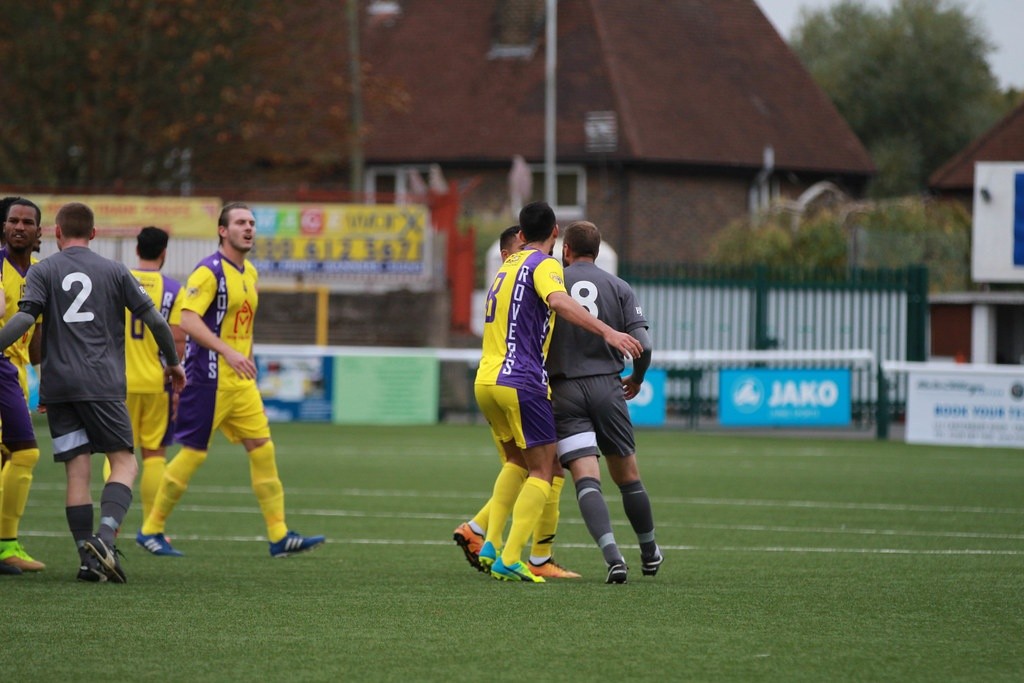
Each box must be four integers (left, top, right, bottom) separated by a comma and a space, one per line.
547, 222, 663, 583
0, 196, 186, 583
136, 201, 325, 557
452, 202, 644, 583
103, 227, 186, 545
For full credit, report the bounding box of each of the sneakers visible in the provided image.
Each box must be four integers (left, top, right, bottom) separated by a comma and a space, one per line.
269, 531, 326, 559
478, 541, 504, 573
75, 561, 113, 582
605, 559, 627, 584
454, 523, 486, 571
0, 540, 45, 572
640, 552, 666, 575
136, 531, 185, 557
83, 534, 129, 584
490, 557, 546, 583
526, 558, 583, 580
0, 561, 22, 575
